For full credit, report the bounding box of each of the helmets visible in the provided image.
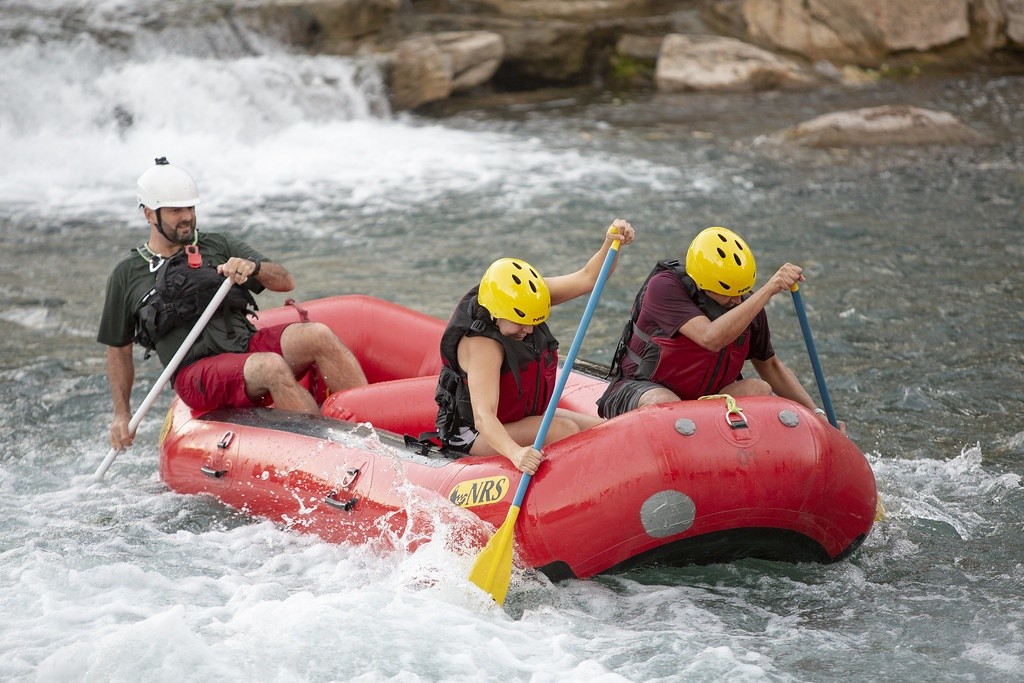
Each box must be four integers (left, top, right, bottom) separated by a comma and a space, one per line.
136, 163, 202, 211
478, 257, 551, 325
685, 225, 758, 296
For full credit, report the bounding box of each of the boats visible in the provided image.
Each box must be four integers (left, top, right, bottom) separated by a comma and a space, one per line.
159, 294, 878, 586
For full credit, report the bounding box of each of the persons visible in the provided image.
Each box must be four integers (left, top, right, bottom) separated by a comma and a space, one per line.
434, 219, 635, 475
595, 227, 845, 437
97, 157, 368, 452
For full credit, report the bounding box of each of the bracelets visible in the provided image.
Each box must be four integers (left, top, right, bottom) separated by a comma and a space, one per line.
812, 407, 825, 416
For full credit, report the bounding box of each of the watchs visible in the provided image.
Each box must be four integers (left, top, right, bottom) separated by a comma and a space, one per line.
247, 257, 260, 277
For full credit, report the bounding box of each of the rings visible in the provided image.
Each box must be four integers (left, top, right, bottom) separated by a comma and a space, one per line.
236, 271, 242, 274
630, 228, 634, 232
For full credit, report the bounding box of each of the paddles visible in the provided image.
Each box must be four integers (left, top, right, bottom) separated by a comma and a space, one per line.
789, 279, 883, 521
92, 263, 247, 485
468, 227, 622, 609
